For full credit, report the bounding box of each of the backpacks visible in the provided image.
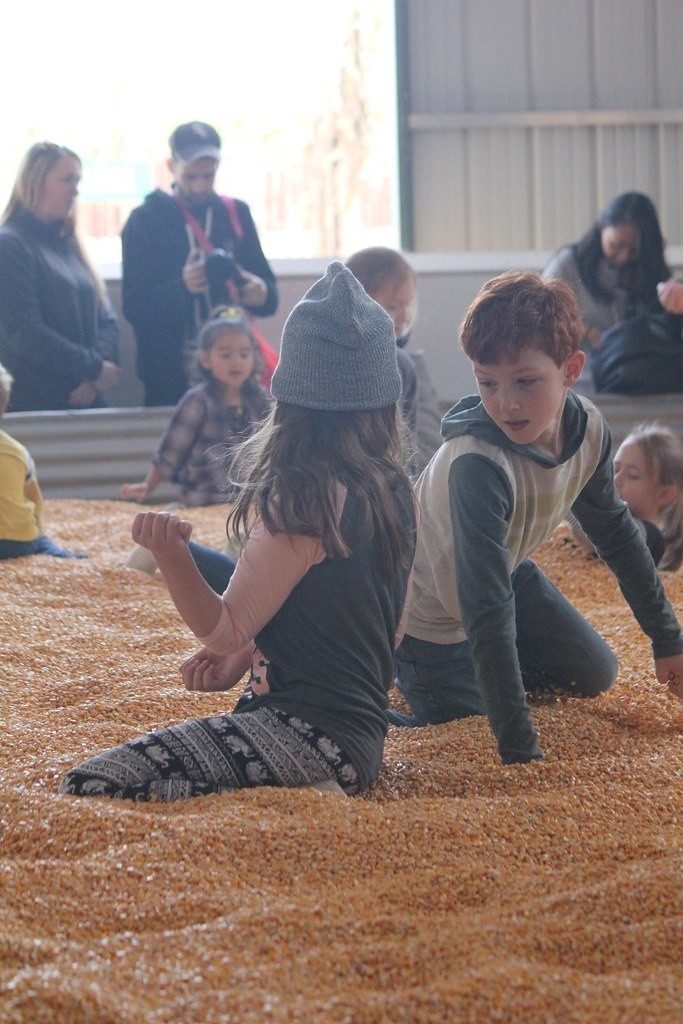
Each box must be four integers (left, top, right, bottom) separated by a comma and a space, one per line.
592, 318, 677, 395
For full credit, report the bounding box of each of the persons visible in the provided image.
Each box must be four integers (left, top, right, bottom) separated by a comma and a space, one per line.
119, 119, 280, 409
0, 140, 124, 412
0, 367, 84, 559
59, 190, 682, 800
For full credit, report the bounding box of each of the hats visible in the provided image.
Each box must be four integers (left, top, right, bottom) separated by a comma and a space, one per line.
169, 121, 221, 160
269, 262, 398, 410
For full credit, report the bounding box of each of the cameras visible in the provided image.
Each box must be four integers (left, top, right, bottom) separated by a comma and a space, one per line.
195, 249, 243, 296
